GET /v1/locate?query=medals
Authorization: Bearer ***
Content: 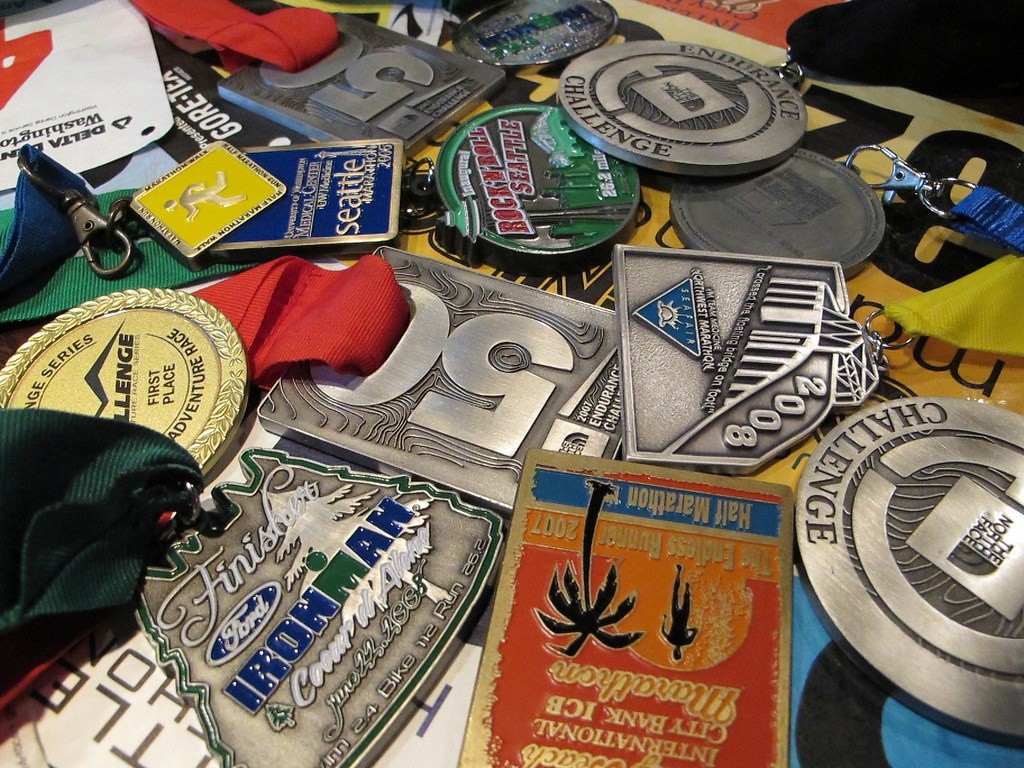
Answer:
[0,0,1024,768]
